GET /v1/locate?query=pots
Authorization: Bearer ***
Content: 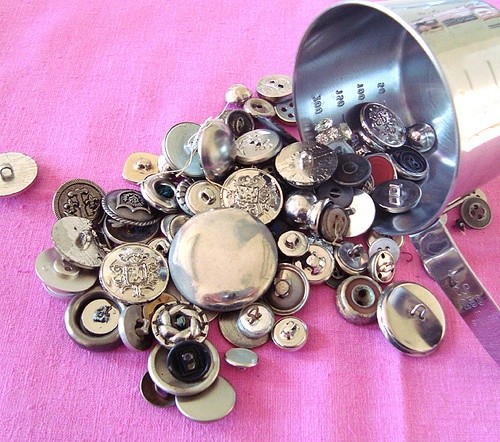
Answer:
[293,0,500,365]
